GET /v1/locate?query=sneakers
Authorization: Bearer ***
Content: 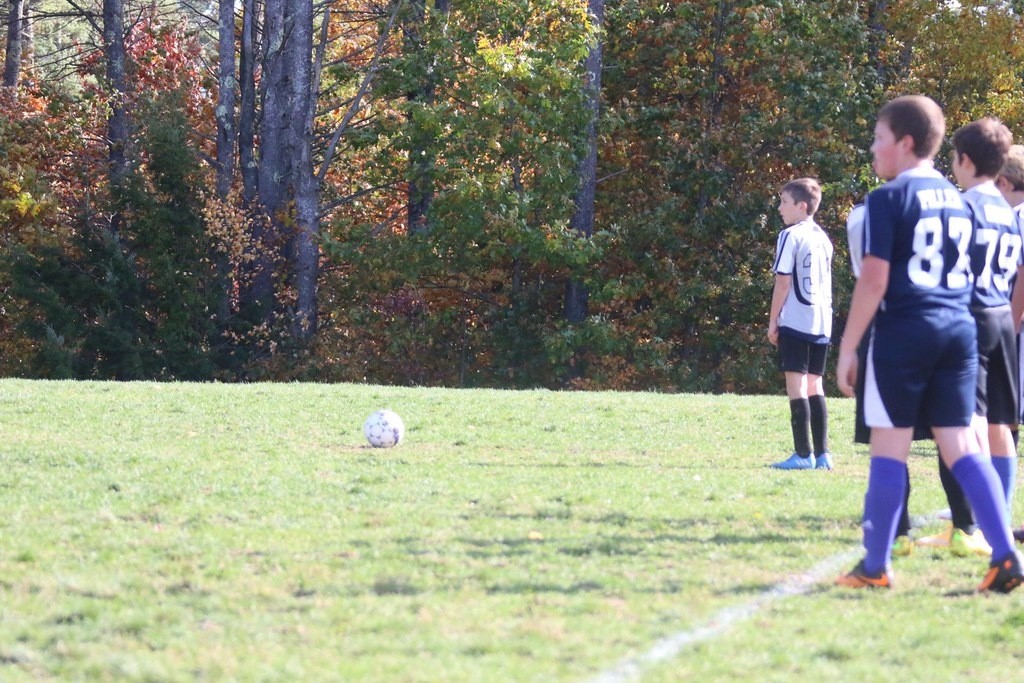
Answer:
[833,558,891,587]
[914,525,993,555]
[770,452,816,468]
[892,535,912,555]
[816,452,831,470]
[974,549,1024,593]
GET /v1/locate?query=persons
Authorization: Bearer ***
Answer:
[844,175,1003,562]
[931,142,1024,545]
[828,93,1024,595]
[763,177,836,472]
[913,114,1024,552]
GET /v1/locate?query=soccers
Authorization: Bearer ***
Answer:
[363,407,407,450]
